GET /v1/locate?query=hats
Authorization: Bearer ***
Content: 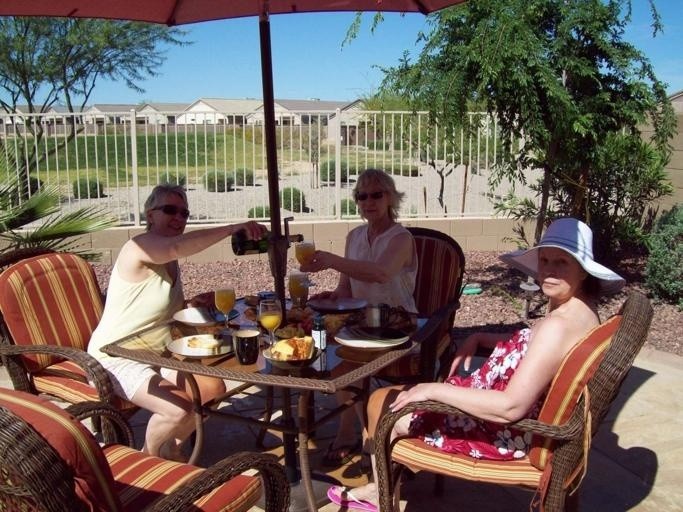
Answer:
[498,217,627,297]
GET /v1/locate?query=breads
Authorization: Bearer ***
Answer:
[271,336,316,362]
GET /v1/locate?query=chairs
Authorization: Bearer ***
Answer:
[373,292,654,512]
[0,385,291,512]
[368,227,465,383]
[0,246,198,449]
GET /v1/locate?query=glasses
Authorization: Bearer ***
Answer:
[355,192,383,200]
[152,205,190,219]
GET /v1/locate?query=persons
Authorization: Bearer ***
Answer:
[297,168,421,461]
[85,184,267,463]
[328,215,635,511]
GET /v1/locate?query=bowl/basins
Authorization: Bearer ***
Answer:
[262,347,324,369]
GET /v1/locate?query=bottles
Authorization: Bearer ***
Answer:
[310,317,327,351]
[231,231,304,256]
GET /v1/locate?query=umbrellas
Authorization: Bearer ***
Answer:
[0,1,461,483]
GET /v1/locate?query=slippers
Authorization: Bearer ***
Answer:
[327,486,378,511]
[320,442,351,465]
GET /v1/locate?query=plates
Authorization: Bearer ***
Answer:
[306,295,367,310]
[172,307,239,326]
[166,333,233,358]
[333,326,409,349]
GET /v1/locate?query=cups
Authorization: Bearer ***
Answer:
[244,295,258,307]
[364,301,383,327]
[288,273,308,306]
[233,330,260,364]
[244,308,256,322]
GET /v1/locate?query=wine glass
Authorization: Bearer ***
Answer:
[294,240,318,288]
[214,288,235,334]
[259,298,282,348]
